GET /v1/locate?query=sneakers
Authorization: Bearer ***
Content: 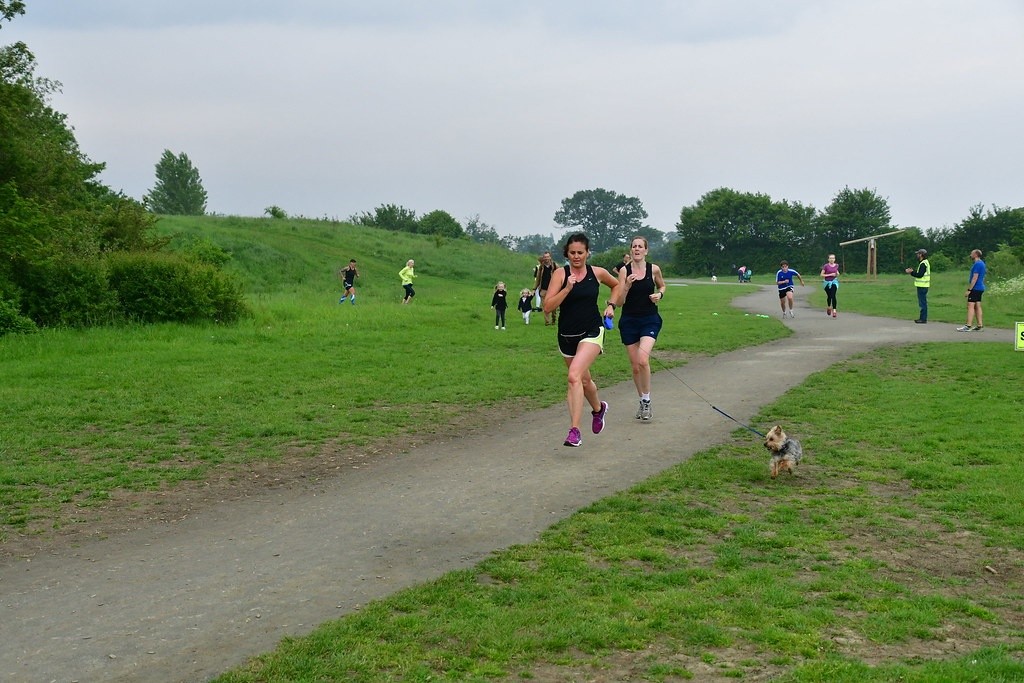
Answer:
[591,401,608,434]
[636,401,642,419]
[641,399,653,420]
[957,325,972,332]
[972,326,984,331]
[564,427,582,447]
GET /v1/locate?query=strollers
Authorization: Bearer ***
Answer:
[742,270,752,283]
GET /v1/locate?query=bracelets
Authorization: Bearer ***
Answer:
[968,288,972,291]
[658,291,664,299]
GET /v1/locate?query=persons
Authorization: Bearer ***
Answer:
[956,250,986,332]
[490,281,508,330]
[544,233,619,447]
[517,288,535,325]
[398,259,418,304]
[775,260,805,319]
[615,237,665,420]
[820,254,840,318]
[532,256,543,309]
[338,259,359,305]
[612,253,630,275]
[905,249,931,323]
[738,266,746,282]
[531,252,559,326]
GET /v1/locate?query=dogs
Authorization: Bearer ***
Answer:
[711,275,717,282]
[764,425,802,478]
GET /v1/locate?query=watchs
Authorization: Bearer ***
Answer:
[609,303,616,309]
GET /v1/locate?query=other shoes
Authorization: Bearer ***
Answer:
[833,312,836,317]
[496,326,499,329]
[789,310,795,318]
[551,321,556,325]
[501,327,506,330]
[545,322,549,325]
[783,311,787,319]
[827,308,831,315]
[915,319,927,323]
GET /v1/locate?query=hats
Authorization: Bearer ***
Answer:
[350,259,356,262]
[915,249,928,256]
